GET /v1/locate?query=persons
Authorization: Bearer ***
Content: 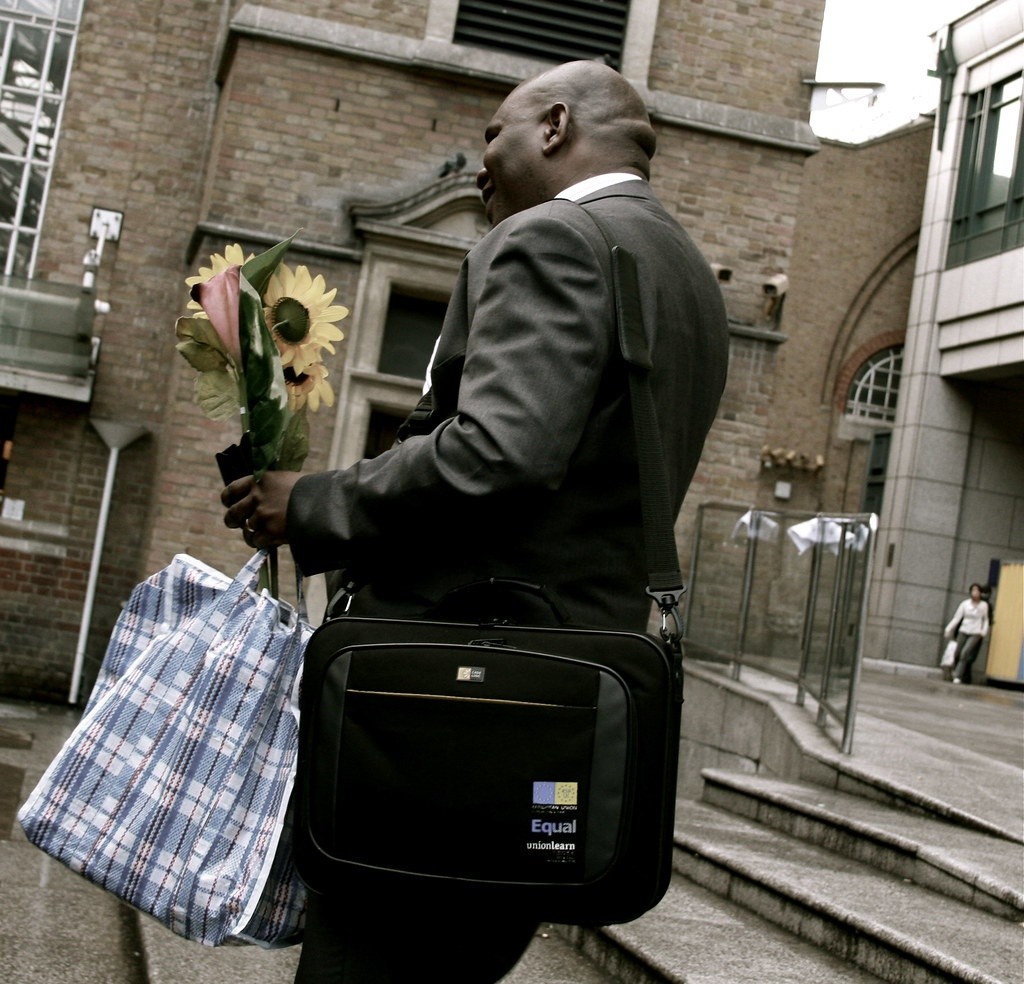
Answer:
[944,583,990,687]
[215,63,728,984]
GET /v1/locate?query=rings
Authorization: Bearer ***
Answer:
[242,519,256,536]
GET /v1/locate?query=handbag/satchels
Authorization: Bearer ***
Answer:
[15,544,329,952]
[289,606,685,930]
[938,636,961,670]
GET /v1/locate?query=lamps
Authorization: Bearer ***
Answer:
[758,440,826,499]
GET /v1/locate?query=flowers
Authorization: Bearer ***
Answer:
[172,229,350,599]
